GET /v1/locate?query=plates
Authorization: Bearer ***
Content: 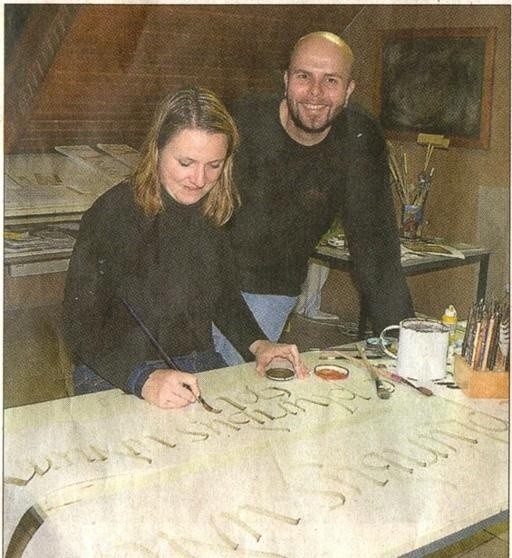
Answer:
[367,337,391,348]
[266,367,294,381]
[314,364,349,380]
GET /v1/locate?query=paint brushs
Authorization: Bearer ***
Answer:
[309,343,433,399]
[385,139,434,205]
[117,292,213,412]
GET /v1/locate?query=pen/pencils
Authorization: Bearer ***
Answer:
[461,298,502,372]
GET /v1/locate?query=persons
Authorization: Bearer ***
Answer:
[213,30,415,366]
[60,87,311,408]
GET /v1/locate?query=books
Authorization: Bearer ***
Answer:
[5,138,140,261]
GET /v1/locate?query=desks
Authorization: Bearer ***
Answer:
[2,330,510,557]
[310,227,493,337]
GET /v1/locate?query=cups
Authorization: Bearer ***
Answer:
[379,318,450,382]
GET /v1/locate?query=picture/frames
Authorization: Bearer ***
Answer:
[370,25,494,154]
